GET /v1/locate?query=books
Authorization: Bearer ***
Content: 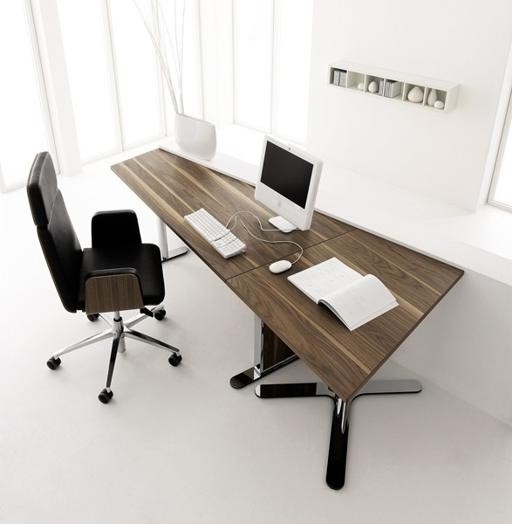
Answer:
[288,255,399,331]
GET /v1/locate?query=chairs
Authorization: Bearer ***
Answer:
[169,112,218,163]
[27,149,183,406]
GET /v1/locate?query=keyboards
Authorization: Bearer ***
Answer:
[184,208,247,260]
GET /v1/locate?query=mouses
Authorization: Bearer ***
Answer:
[269,260,292,273]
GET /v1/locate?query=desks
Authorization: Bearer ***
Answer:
[111,147,464,491]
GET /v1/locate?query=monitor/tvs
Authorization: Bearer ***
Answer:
[255,135,323,234]
